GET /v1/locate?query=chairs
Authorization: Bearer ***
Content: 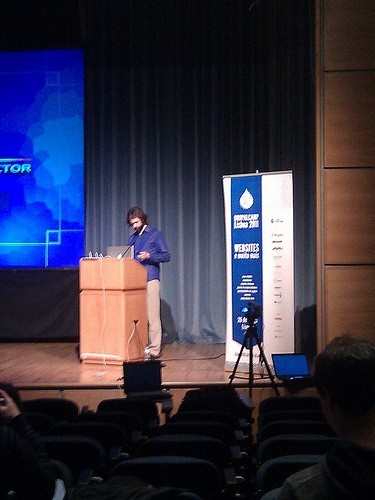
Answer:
[14,391,341,500]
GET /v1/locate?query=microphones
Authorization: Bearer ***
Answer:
[121,242,135,259]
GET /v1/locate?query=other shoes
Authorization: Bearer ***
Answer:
[144,353,157,360]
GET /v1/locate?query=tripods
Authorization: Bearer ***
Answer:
[228,318,280,399]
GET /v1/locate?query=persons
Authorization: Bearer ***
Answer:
[0,381,56,500]
[67,475,185,500]
[276,333,375,500]
[126,207,171,357]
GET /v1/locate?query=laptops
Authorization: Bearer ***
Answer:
[123,360,173,399]
[108,246,134,259]
[271,353,314,381]
